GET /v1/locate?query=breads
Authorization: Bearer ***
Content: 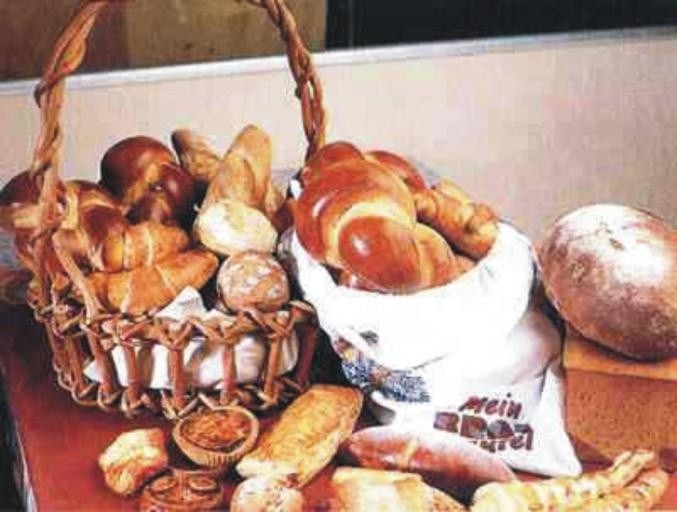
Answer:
[173,405,258,467]
[329,465,464,511]
[539,204,676,360]
[0,124,290,322]
[140,472,224,511]
[230,476,307,512]
[293,142,495,296]
[236,383,363,487]
[471,447,669,512]
[98,428,166,495]
[344,424,516,503]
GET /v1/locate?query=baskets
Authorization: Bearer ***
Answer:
[1,0,328,420]
